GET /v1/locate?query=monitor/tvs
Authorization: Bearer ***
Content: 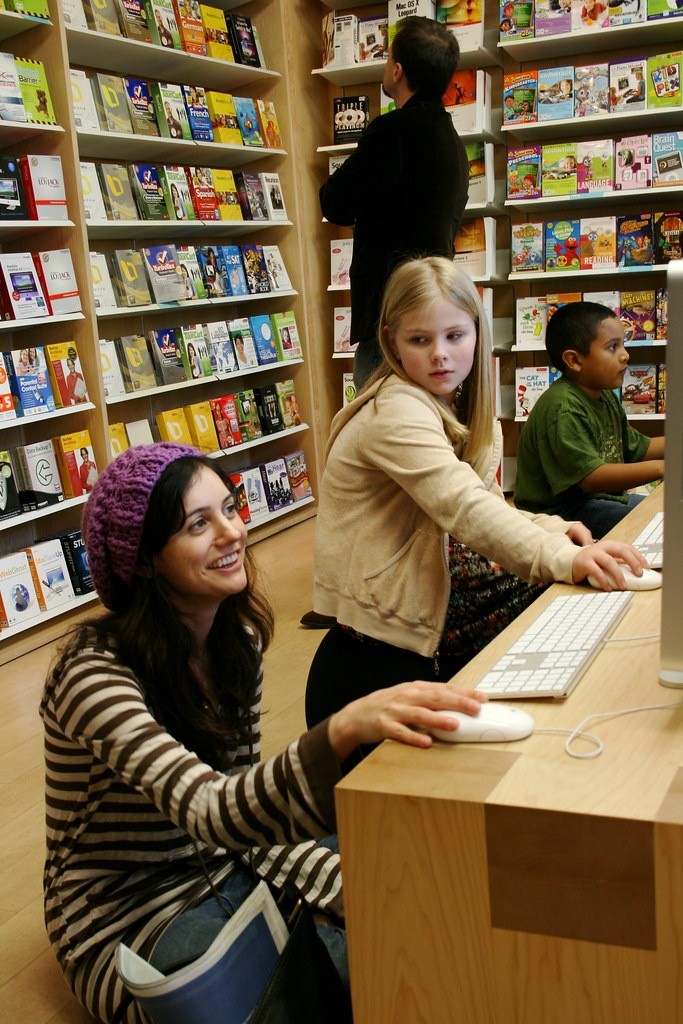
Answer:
[655,259,683,691]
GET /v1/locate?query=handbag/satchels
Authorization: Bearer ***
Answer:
[112,860,352,1024]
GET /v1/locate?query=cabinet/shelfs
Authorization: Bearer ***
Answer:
[311,0,683,504]
[0,0,324,661]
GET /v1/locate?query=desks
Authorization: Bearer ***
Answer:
[335,481,683,1023]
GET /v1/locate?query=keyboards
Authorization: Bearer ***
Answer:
[477,589,636,699]
[613,512,666,569]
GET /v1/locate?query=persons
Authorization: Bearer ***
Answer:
[194,167,214,189]
[188,85,202,108]
[80,446,99,493]
[509,299,667,539]
[153,8,174,49]
[179,264,198,300]
[272,185,277,208]
[280,327,292,349]
[169,342,183,363]
[233,332,250,367]
[38,443,488,1024]
[27,348,40,370]
[66,357,89,404]
[625,72,645,104]
[558,78,572,98]
[283,398,296,425]
[609,87,621,106]
[319,13,471,405]
[166,101,183,138]
[170,184,189,220]
[313,251,648,660]
[205,246,226,296]
[289,393,299,415]
[18,349,30,376]
[186,343,204,378]
[246,393,261,431]
[563,155,577,170]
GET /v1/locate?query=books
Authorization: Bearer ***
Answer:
[501,0,683,424]
[0,0,320,632]
[317,0,487,408]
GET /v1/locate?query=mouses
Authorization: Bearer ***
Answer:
[587,561,662,592]
[427,701,537,742]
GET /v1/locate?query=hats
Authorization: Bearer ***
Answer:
[80,442,205,612]
